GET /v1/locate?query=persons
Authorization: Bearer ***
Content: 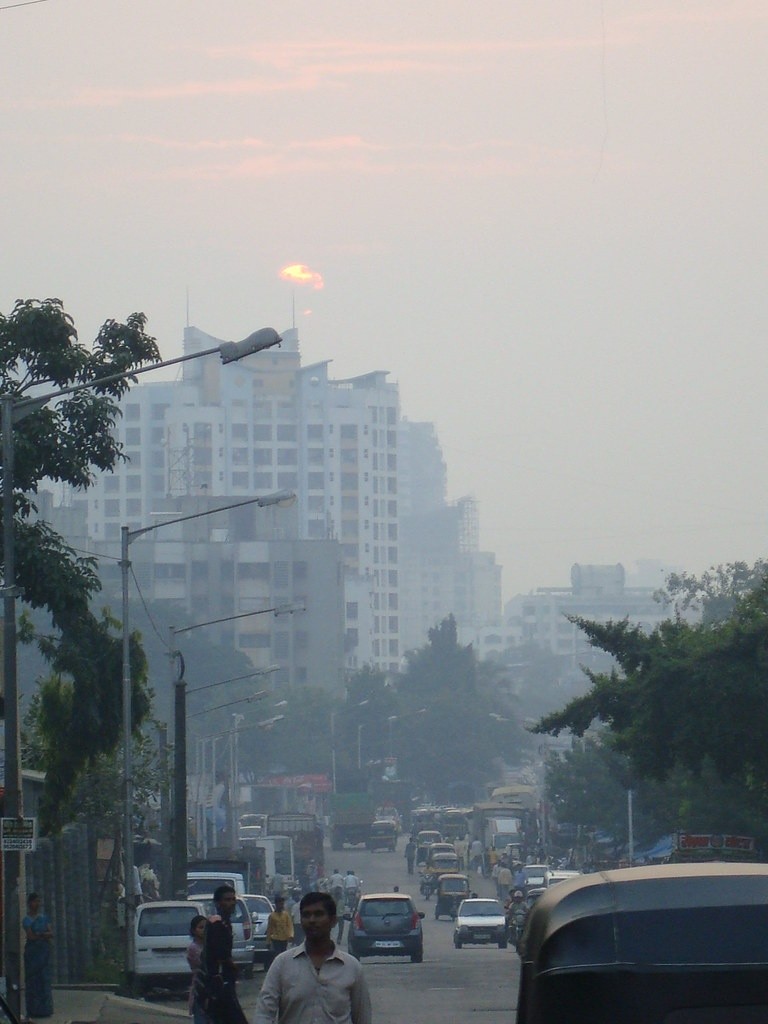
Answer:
[318,869,358,911]
[252,893,374,1024]
[185,884,250,1024]
[505,889,529,932]
[521,840,598,875]
[22,893,54,1016]
[265,893,304,952]
[453,829,527,901]
[404,837,416,874]
[265,869,286,899]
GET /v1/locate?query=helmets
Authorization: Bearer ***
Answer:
[514,891,523,902]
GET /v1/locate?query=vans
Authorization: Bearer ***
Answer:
[523,864,583,906]
[453,898,507,949]
[133,901,209,975]
[186,893,258,966]
[239,894,275,954]
[186,872,245,894]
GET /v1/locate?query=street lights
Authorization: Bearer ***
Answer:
[487,713,585,751]
[2,324,284,1022]
[509,649,634,861]
[169,602,305,863]
[118,490,298,991]
[332,698,427,792]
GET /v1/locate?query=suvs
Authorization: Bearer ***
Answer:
[343,893,426,963]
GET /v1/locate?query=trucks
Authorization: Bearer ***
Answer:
[473,784,539,847]
[331,792,375,851]
[267,812,325,876]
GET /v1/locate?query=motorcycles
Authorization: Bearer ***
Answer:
[368,819,397,854]
[411,806,473,920]
[516,862,768,1024]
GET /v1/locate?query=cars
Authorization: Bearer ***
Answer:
[376,807,402,823]
